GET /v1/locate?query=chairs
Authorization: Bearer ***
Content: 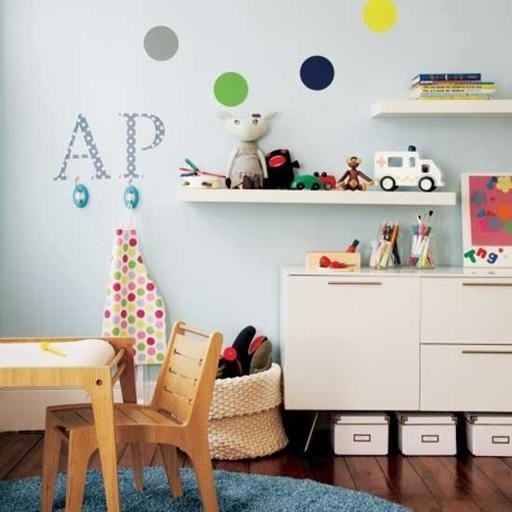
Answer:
[40,320,224,512]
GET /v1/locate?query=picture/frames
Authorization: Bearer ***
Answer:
[460,173,512,266]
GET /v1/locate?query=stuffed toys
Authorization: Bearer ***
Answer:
[262,150,300,188]
[336,155,374,191]
[216,110,277,189]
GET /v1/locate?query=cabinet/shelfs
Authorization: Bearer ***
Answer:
[279,265,512,413]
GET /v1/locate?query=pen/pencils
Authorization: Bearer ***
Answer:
[41,341,67,358]
[411,208,435,270]
[373,218,401,269]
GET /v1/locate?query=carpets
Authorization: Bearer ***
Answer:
[0,464,417,512]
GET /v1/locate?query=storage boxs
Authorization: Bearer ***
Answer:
[465,413,511,458]
[328,414,390,457]
[397,414,459,457]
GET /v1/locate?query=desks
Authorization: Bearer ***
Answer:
[1,334,144,512]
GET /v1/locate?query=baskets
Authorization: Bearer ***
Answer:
[208,363,290,460]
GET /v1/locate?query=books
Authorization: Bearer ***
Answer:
[410,73,496,101]
